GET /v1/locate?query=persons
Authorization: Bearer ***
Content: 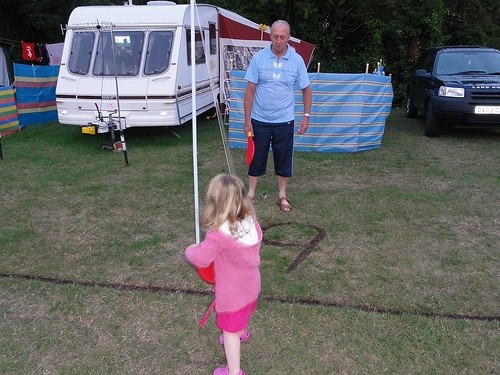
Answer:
[244,20,312,212]
[184,173,264,375]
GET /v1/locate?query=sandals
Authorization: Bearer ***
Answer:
[213,367,245,375]
[217,331,250,344]
[277,197,292,212]
[248,194,254,201]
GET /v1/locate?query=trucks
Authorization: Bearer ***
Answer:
[55,1,218,128]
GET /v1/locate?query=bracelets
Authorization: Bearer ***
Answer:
[304,113,310,118]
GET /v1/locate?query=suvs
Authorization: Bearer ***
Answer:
[406,45,500,138]
